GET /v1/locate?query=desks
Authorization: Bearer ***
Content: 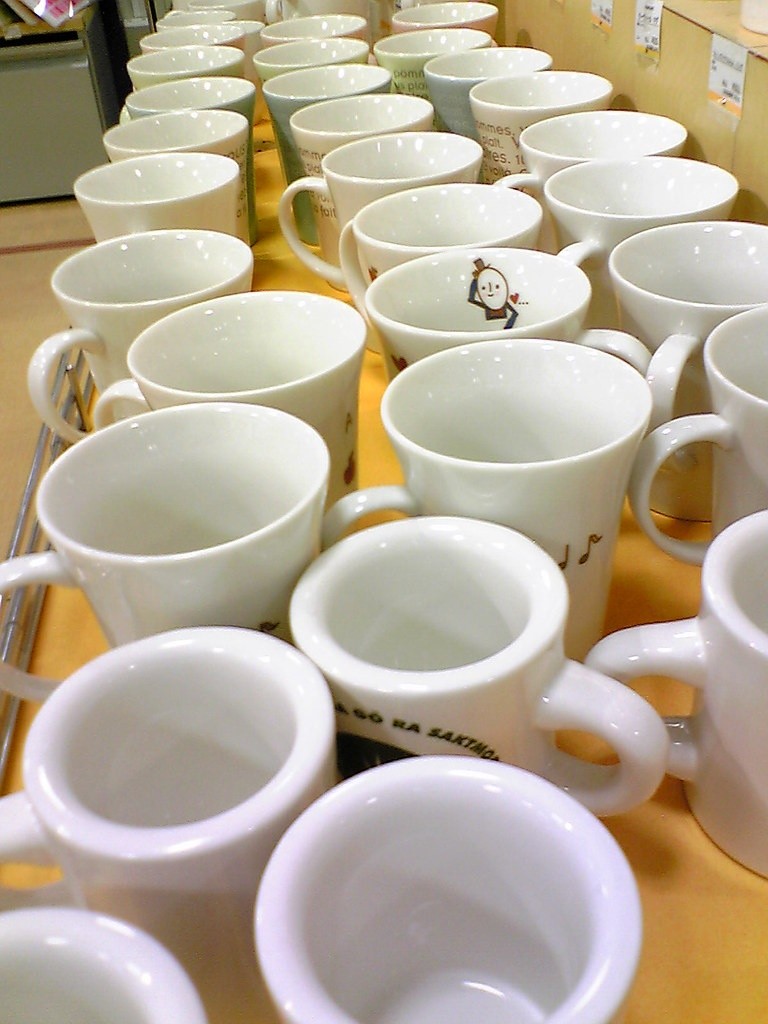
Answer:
[0,77,768,1024]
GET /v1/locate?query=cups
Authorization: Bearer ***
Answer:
[0,628,339,1024]
[0,400,330,702]
[627,306,768,567]
[287,515,671,818]
[587,508,768,880]
[252,754,642,1024]
[26,0,768,506]
[323,339,654,665]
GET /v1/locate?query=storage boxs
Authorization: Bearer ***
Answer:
[502,0,768,226]
